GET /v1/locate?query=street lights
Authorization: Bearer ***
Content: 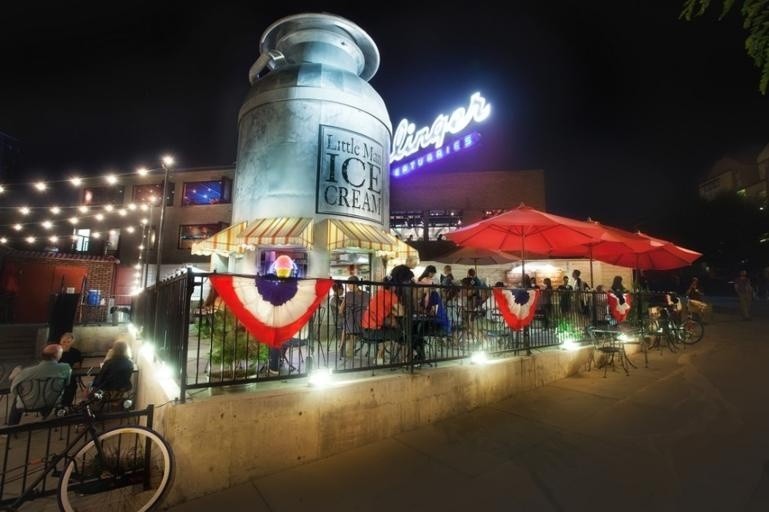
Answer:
[136,154,174,292]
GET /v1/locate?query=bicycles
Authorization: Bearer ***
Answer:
[635,292,705,350]
[0,390,176,512]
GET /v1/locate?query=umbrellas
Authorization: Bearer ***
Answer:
[596,227,705,328]
[548,216,664,330]
[440,201,625,358]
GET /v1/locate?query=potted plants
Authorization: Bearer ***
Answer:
[192,308,269,397]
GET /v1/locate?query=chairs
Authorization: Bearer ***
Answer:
[279,285,686,386]
[1,346,142,441]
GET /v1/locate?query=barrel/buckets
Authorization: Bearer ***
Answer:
[87,290,101,305]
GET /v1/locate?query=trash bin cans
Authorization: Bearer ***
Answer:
[110,306,129,325]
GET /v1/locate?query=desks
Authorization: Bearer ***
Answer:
[74,302,106,326]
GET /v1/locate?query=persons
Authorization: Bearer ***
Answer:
[407,234,412,242]
[9,333,133,426]
[687,277,699,301]
[524,269,627,327]
[331,264,482,365]
[734,268,753,320]
[438,234,442,240]
